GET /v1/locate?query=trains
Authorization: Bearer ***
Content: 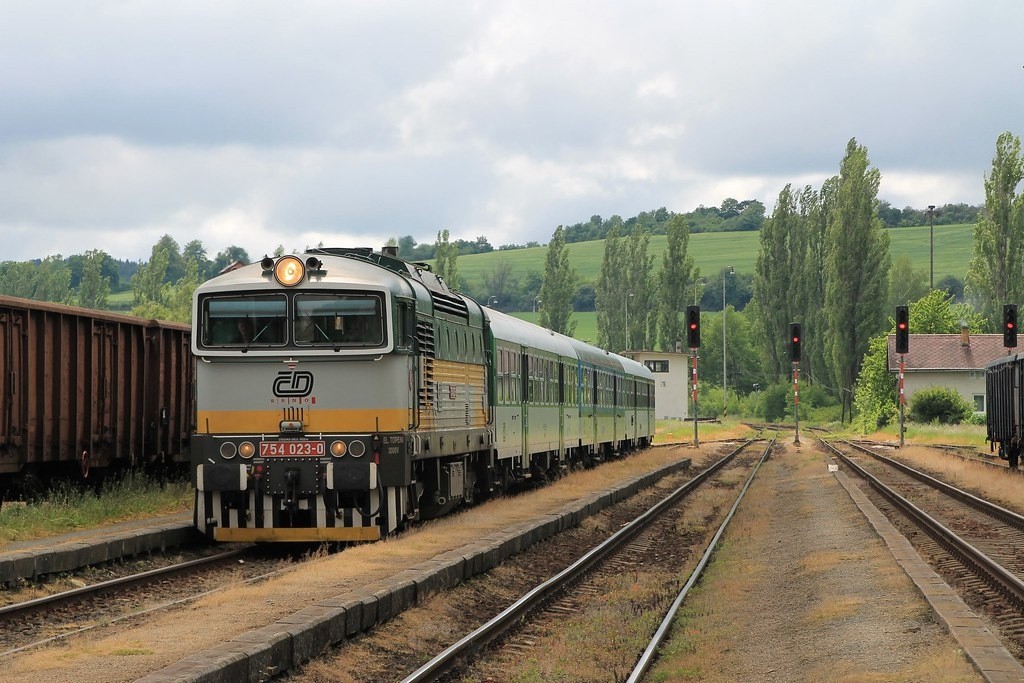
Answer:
[188,246,658,555]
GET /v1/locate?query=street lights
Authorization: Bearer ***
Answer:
[625,287,637,351]
[695,277,708,305]
[722,264,736,424]
[533,295,543,324]
[487,294,501,308]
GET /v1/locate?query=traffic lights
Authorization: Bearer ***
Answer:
[790,323,802,363]
[895,306,908,353]
[1003,303,1018,348]
[686,306,701,347]
